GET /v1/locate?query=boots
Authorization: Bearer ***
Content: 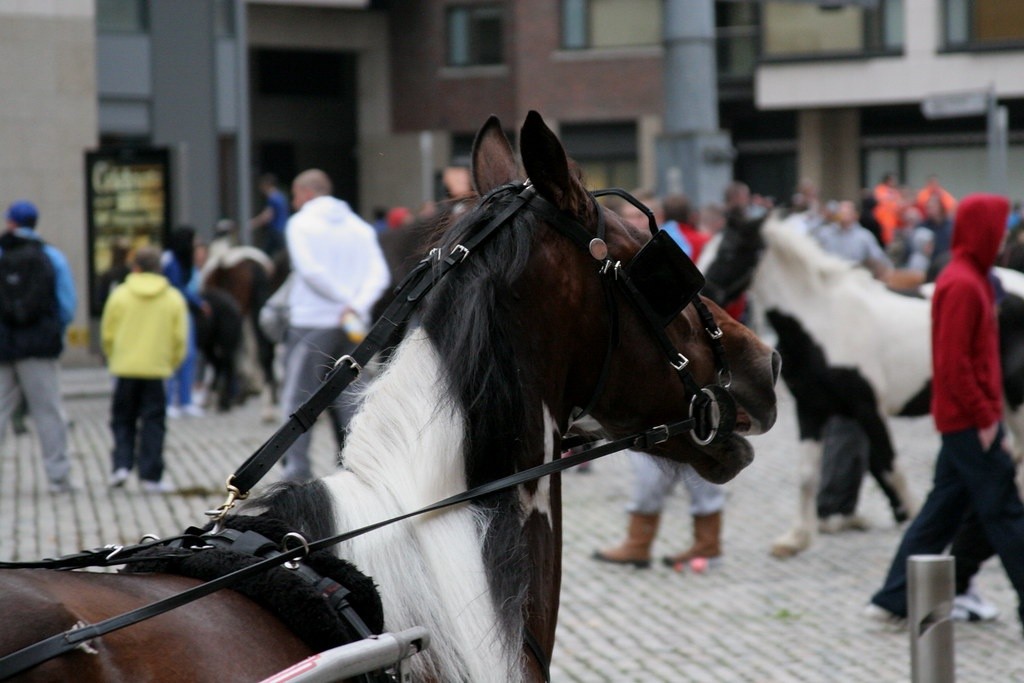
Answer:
[661,512,721,566]
[593,510,661,570]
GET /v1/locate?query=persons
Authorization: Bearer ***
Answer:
[99,246,188,494]
[98,168,1024,637]
[276,168,393,485]
[0,198,80,496]
[865,193,1024,636]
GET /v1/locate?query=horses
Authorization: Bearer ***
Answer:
[0,107,784,682]
[694,200,1024,559]
[180,240,288,416]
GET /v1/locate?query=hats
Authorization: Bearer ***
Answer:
[8,202,39,230]
[135,246,161,274]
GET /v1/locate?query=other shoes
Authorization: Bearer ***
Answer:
[105,469,127,489]
[952,593,999,626]
[862,602,908,632]
[137,477,172,492]
[167,406,198,418]
[51,479,81,497]
